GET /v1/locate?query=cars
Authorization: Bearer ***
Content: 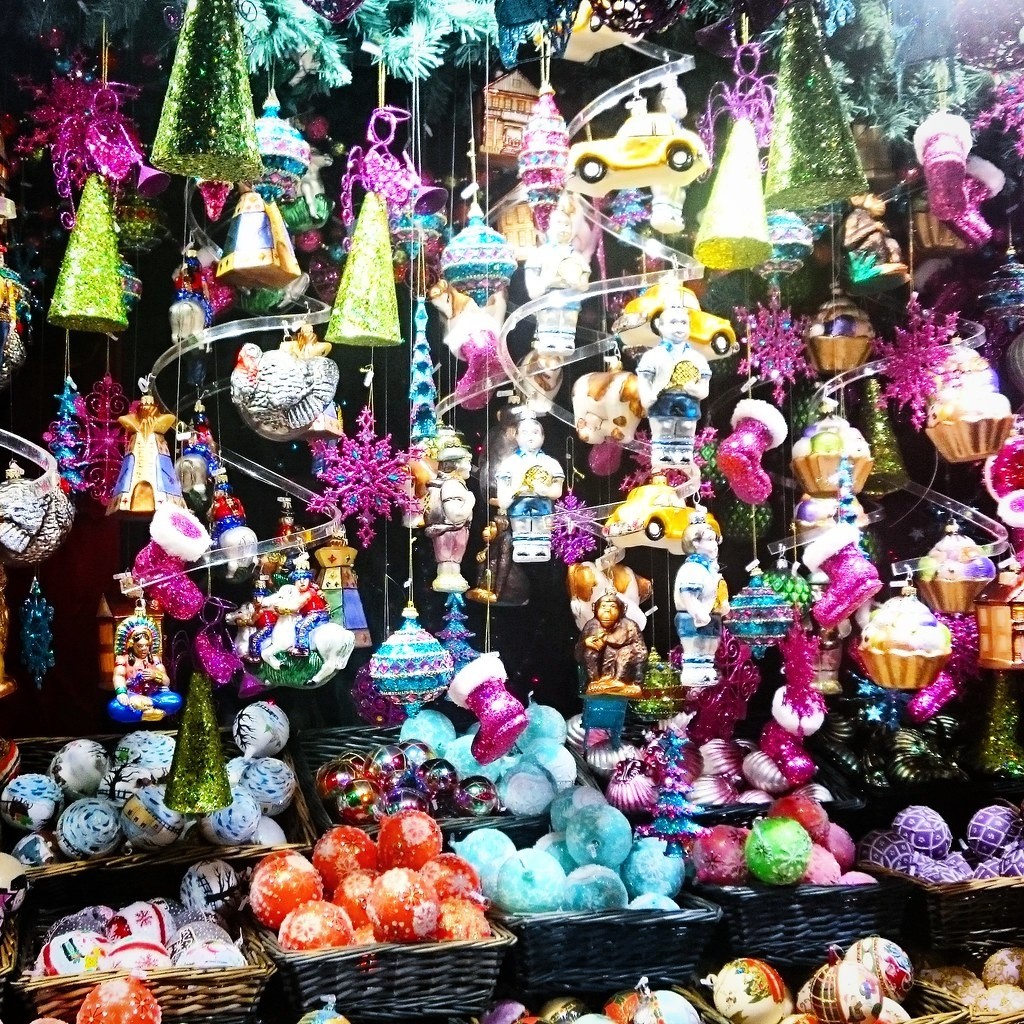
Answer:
[605,477,727,553]
[570,111,703,182]
[615,279,743,360]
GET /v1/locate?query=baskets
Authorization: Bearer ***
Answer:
[0,725,1024,1024]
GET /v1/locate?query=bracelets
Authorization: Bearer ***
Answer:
[116,687,126,694]
[154,673,162,683]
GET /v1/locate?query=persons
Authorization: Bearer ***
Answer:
[109,628,182,722]
[430,446,475,593]
[673,523,731,688]
[184,413,218,482]
[639,308,713,467]
[273,570,330,657]
[173,257,212,329]
[237,588,278,664]
[523,210,591,356]
[278,509,299,571]
[206,482,245,550]
[498,417,565,561]
